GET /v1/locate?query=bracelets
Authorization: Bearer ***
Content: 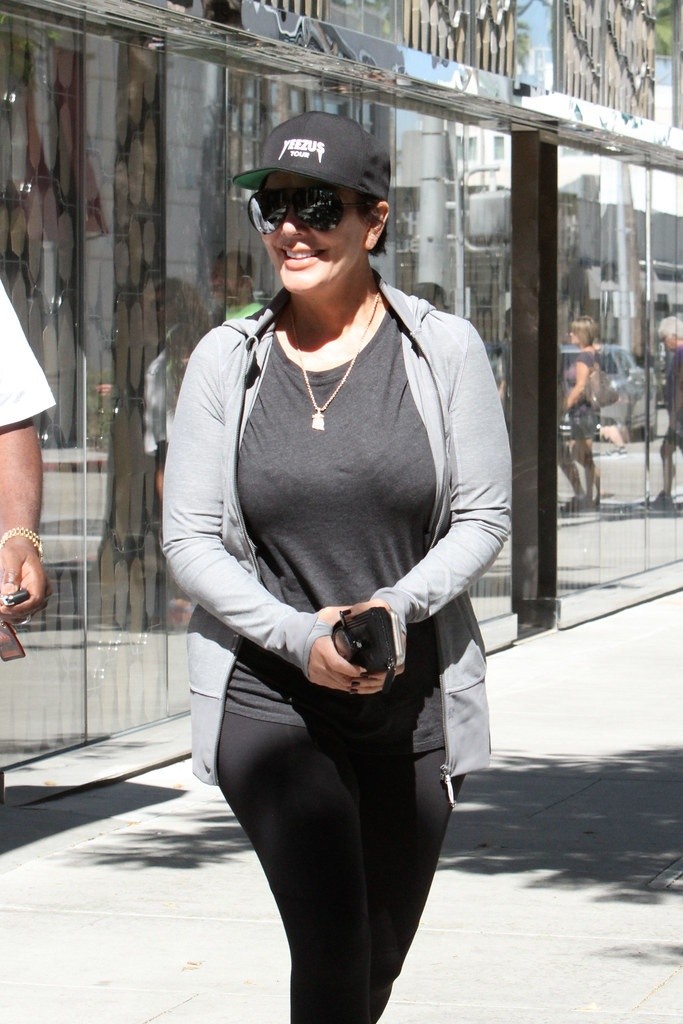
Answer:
[0,526,43,563]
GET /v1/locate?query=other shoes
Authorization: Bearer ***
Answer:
[559,493,596,513]
[639,492,675,512]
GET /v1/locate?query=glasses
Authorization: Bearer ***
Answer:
[142,295,172,320]
[332,610,356,664]
[248,188,375,235]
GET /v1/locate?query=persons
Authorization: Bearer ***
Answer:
[0,275,56,627]
[497,306,627,510]
[212,248,265,324]
[649,315,683,511]
[141,324,198,628]
[164,112,511,1024]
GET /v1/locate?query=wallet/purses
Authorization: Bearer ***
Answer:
[333,606,396,684]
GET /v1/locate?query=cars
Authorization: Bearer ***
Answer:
[558,341,658,449]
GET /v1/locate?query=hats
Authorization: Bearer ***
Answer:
[232,111,391,201]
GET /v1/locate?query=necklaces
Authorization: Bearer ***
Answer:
[289,293,380,431]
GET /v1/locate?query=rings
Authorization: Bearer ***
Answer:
[26,614,31,623]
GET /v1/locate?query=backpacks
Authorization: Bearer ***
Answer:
[585,368,620,411]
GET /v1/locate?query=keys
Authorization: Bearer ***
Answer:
[0,590,29,663]
